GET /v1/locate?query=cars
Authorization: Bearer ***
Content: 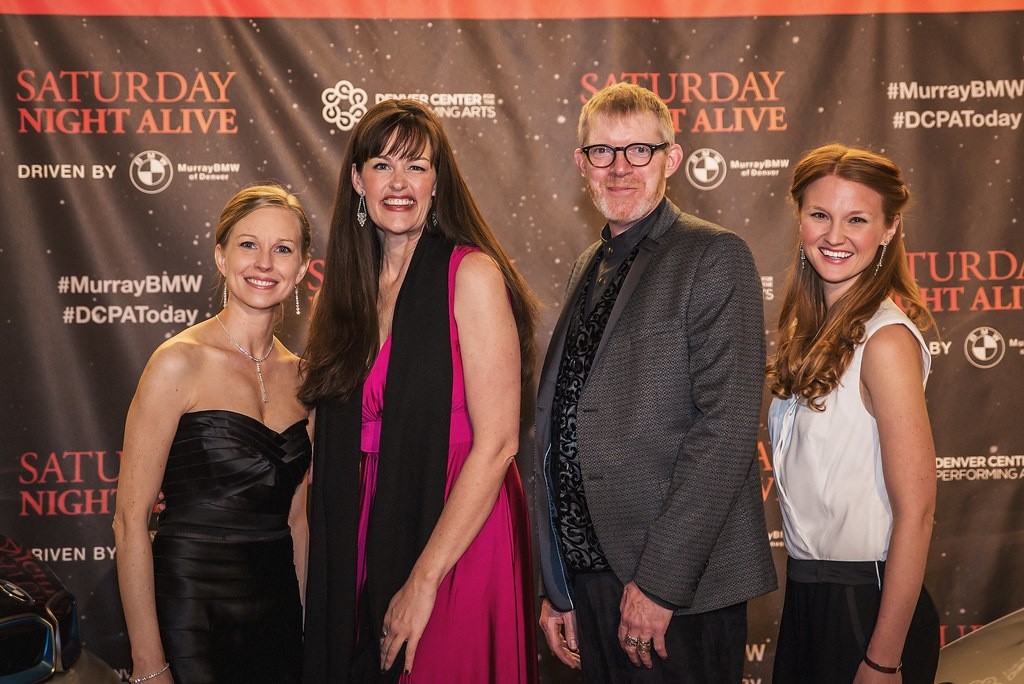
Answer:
[0,534,126,684]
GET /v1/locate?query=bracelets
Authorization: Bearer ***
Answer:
[863,653,902,674]
[129,662,171,684]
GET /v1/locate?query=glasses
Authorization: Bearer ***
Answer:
[580,141,669,168]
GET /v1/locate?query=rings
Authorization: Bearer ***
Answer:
[637,642,651,653]
[624,634,638,647]
[379,630,388,640]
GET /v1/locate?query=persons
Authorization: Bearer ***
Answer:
[290,98,539,684]
[111,182,315,683]
[757,142,943,683]
[532,83,780,684]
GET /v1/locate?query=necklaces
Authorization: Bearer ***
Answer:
[214,314,275,405]
